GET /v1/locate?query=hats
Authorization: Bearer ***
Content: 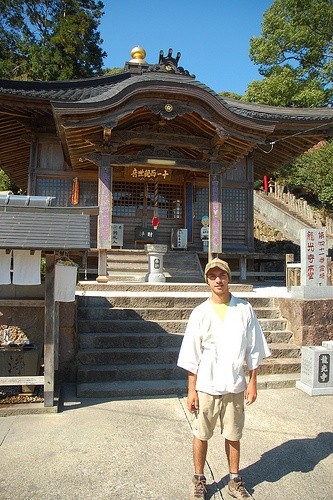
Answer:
[204,258,231,282]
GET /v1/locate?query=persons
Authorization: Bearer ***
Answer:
[175,257,273,500]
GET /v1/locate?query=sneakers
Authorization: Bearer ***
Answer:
[191,475,206,500]
[228,476,255,500]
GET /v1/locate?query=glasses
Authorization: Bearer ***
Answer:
[208,273,227,281]
